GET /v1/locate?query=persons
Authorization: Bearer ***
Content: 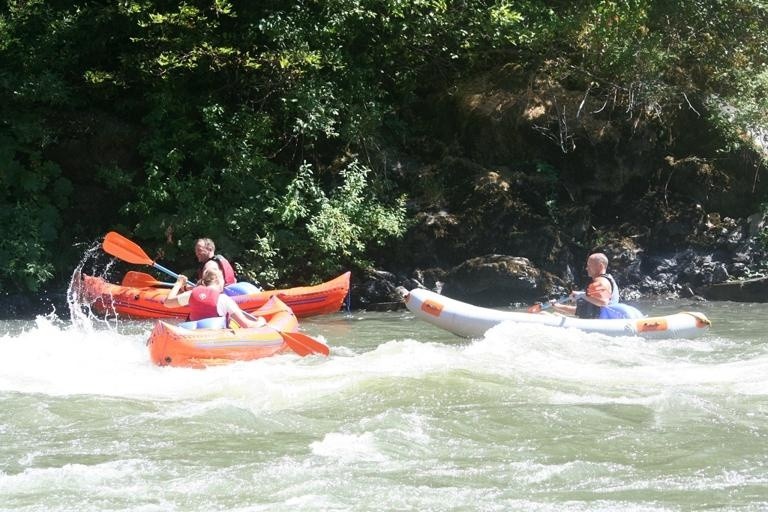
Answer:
[195,238,238,286]
[164,268,266,330]
[539,253,619,319]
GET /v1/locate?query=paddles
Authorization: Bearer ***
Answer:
[240,310,328,359]
[102,231,198,289]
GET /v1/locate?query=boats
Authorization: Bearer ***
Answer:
[82,269,351,321]
[403,286,712,343]
[147,295,300,368]
